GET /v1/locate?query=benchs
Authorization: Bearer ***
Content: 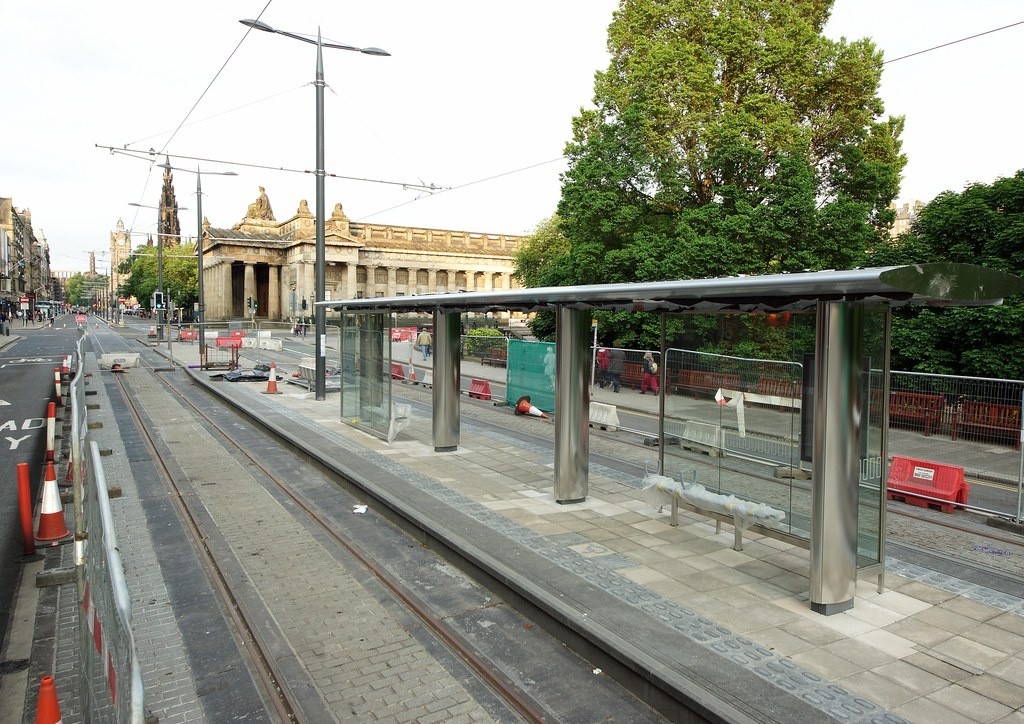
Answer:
[641,459,785,551]
[363,400,408,439]
[481,349,1022,452]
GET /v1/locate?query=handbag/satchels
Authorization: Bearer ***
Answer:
[297,327,302,331]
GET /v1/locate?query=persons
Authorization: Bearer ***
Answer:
[543,347,557,390]
[593,342,625,393]
[246,185,276,220]
[0,307,55,326]
[639,352,659,395]
[289,313,308,337]
[417,328,432,361]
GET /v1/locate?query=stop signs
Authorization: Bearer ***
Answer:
[21,297,29,303]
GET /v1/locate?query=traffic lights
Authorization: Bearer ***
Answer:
[302,300,306,309]
[247,297,251,308]
[254,301,257,308]
[154,292,163,309]
[6,301,11,307]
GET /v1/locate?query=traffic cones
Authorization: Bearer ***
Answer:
[34,461,70,541]
[148,323,156,338]
[514,396,549,419]
[408,359,416,381]
[63,323,67,329]
[111,364,128,373]
[34,676,63,724]
[261,361,283,394]
[95,322,99,329]
[62,355,70,374]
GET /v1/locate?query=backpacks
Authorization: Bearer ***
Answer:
[646,358,657,374]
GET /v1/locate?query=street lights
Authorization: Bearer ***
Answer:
[129,203,189,339]
[240,19,392,401]
[156,163,239,354]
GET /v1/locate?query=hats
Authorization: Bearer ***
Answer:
[422,328,427,330]
[547,347,552,351]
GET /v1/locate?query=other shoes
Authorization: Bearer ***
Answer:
[608,381,613,389]
[614,386,620,393]
[639,392,645,394]
[426,355,427,356]
[655,390,658,396]
[597,386,605,390]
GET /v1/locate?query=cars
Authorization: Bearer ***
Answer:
[79,308,86,315]
